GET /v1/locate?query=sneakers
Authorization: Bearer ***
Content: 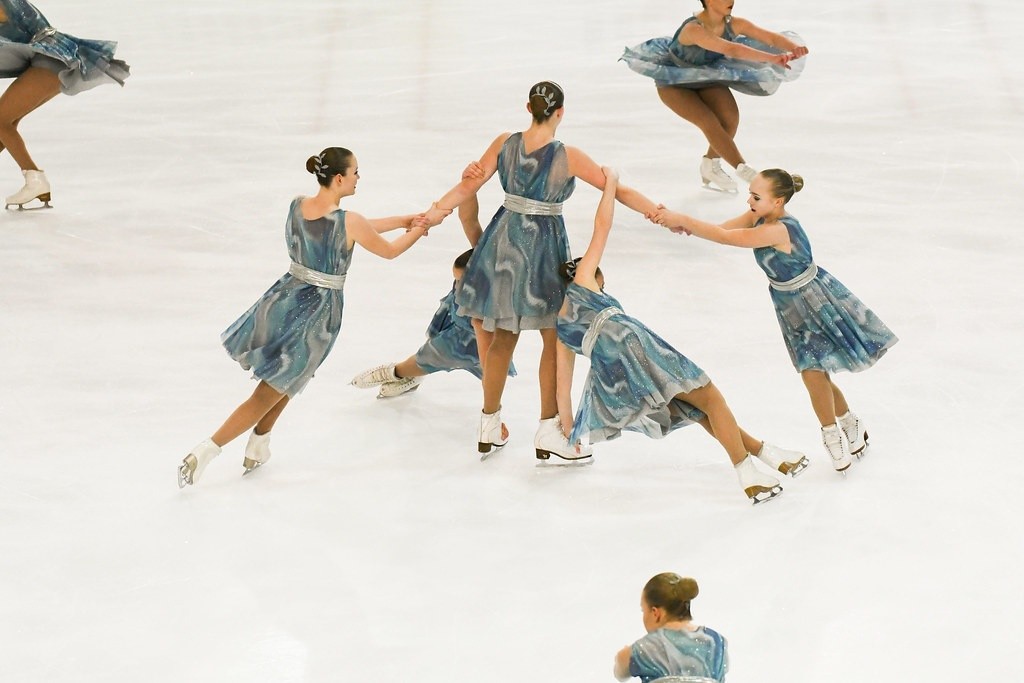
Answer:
[700,155,757,194]
[347,363,424,399]
[243,427,270,475]
[734,452,783,504]
[6,169,51,210]
[534,413,592,460]
[821,424,851,476]
[479,405,509,452]
[178,437,221,488]
[835,409,868,459]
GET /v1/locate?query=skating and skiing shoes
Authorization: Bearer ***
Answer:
[757,441,812,477]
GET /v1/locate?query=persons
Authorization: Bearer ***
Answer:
[458,82,691,466]
[179,147,452,488]
[347,161,517,400]
[617,0,808,193]
[556,166,809,505]
[0,0,130,211]
[645,168,898,476]
[614,572,729,683]
